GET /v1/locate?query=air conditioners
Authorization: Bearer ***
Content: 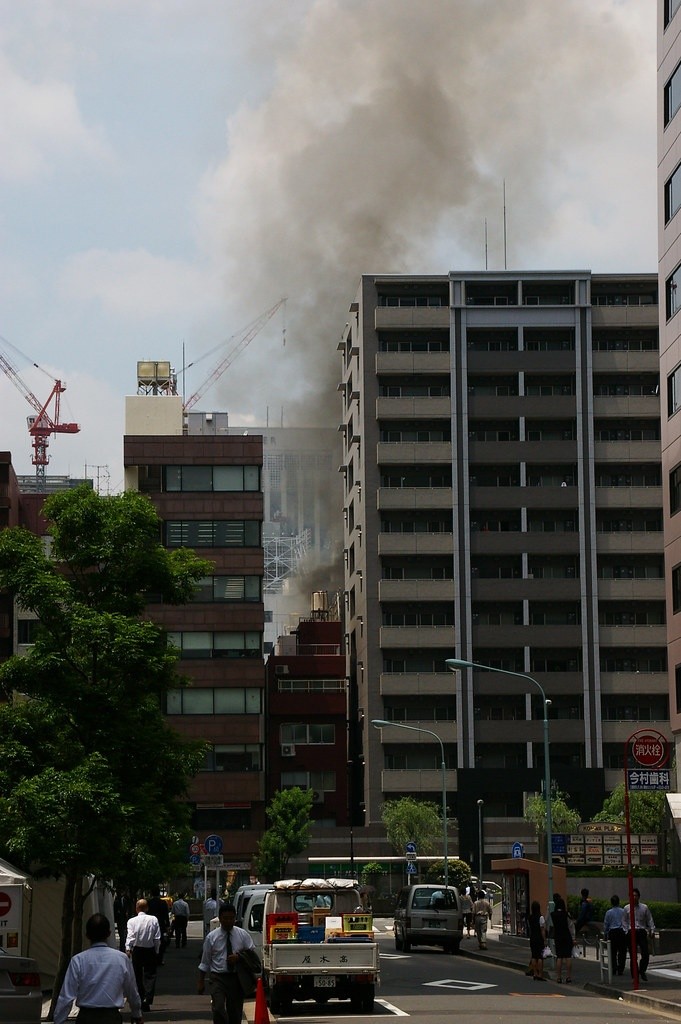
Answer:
[312,790,324,802]
[275,665,288,674]
[281,744,295,756]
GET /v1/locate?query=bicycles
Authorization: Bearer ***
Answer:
[570,909,600,946]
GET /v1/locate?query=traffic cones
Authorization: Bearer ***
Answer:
[254,977,270,1024]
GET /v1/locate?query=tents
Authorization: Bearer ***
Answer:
[0,859,35,957]
[26,873,114,977]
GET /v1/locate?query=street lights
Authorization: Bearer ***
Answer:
[370,720,448,886]
[476,799,484,890]
[444,659,556,956]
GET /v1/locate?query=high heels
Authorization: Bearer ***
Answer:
[537,976,547,981]
[533,975,538,980]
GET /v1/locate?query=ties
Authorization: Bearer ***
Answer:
[226,932,235,972]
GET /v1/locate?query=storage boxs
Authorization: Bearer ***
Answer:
[266,911,375,943]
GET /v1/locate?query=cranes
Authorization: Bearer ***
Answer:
[138,295,289,414]
[0,335,82,494]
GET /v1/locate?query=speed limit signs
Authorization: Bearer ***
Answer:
[190,844,200,854]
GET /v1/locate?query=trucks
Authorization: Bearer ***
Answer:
[261,887,380,1015]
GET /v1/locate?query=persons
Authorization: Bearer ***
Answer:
[470,887,477,904]
[459,888,475,938]
[198,905,256,1024]
[548,893,576,983]
[528,902,547,981]
[172,893,190,948]
[55,913,144,1024]
[125,899,161,1012]
[207,889,224,909]
[604,895,628,975]
[148,887,170,966]
[574,889,594,933]
[431,891,445,904]
[114,891,134,951]
[473,891,493,949]
[622,888,656,981]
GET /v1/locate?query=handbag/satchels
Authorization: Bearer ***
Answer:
[542,946,554,958]
[571,945,581,958]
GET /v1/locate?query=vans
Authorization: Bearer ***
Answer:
[232,885,275,971]
[392,884,464,953]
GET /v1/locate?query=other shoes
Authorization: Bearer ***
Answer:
[479,943,488,950]
[142,1002,150,1012]
[639,969,648,981]
[566,977,571,983]
[618,970,623,975]
[557,978,562,983]
[612,967,617,975]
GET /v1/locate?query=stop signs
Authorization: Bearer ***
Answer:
[0,892,12,917]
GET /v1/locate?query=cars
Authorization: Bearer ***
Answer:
[0,948,42,1024]
[437,874,502,892]
